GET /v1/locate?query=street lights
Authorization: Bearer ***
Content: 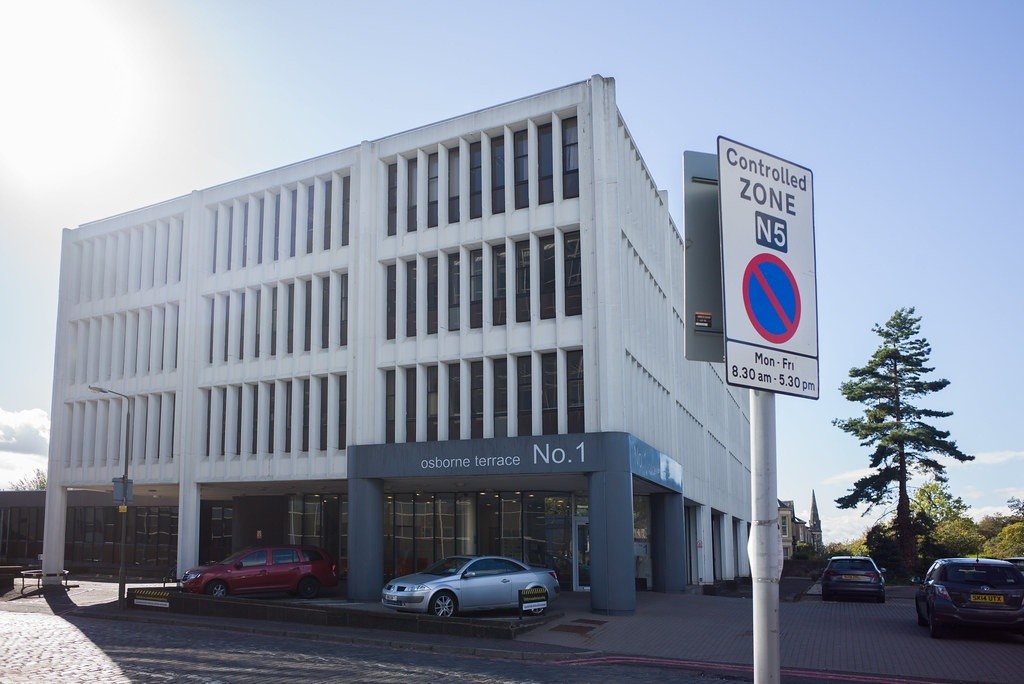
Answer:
[89,385,130,609]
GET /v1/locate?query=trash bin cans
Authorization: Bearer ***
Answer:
[635,577,647,592]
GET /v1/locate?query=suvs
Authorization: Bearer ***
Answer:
[179,545,337,598]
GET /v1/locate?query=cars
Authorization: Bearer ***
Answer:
[910,557,1024,639]
[822,555,885,604]
[503,550,591,591]
[381,556,562,617]
